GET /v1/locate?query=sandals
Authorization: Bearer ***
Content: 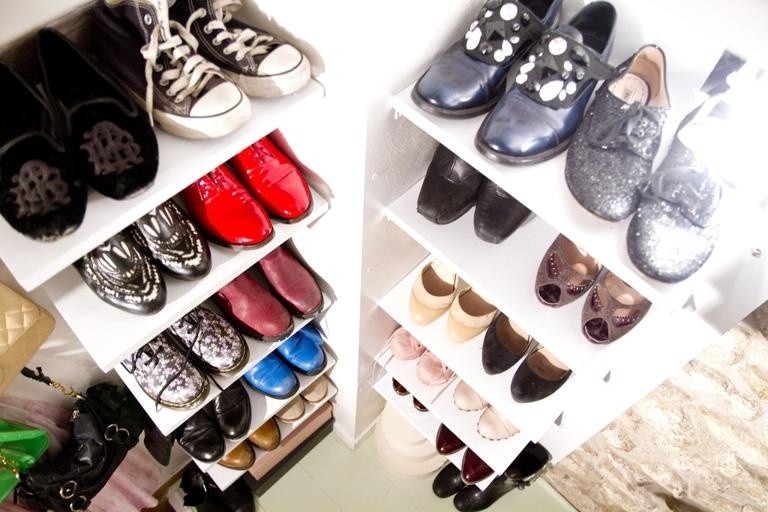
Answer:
[406,259,573,405]
[533,231,653,347]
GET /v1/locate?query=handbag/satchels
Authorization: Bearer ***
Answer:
[0,365,149,512]
[0,279,56,399]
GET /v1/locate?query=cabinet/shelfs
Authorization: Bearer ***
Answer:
[372,50,754,490]
[1,50,339,490]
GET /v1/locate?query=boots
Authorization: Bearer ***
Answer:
[431,439,550,512]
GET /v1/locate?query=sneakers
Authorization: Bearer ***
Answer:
[207,244,325,344]
[119,304,250,412]
[0,25,160,244]
[273,375,329,425]
[388,326,523,443]
[73,0,313,142]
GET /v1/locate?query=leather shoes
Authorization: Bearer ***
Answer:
[434,422,496,487]
[215,416,281,471]
[242,322,327,400]
[390,376,431,413]
[69,196,213,317]
[414,141,534,245]
[408,0,620,169]
[174,134,314,251]
[169,377,252,465]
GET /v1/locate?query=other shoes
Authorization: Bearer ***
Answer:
[564,44,671,221]
[627,94,744,283]
